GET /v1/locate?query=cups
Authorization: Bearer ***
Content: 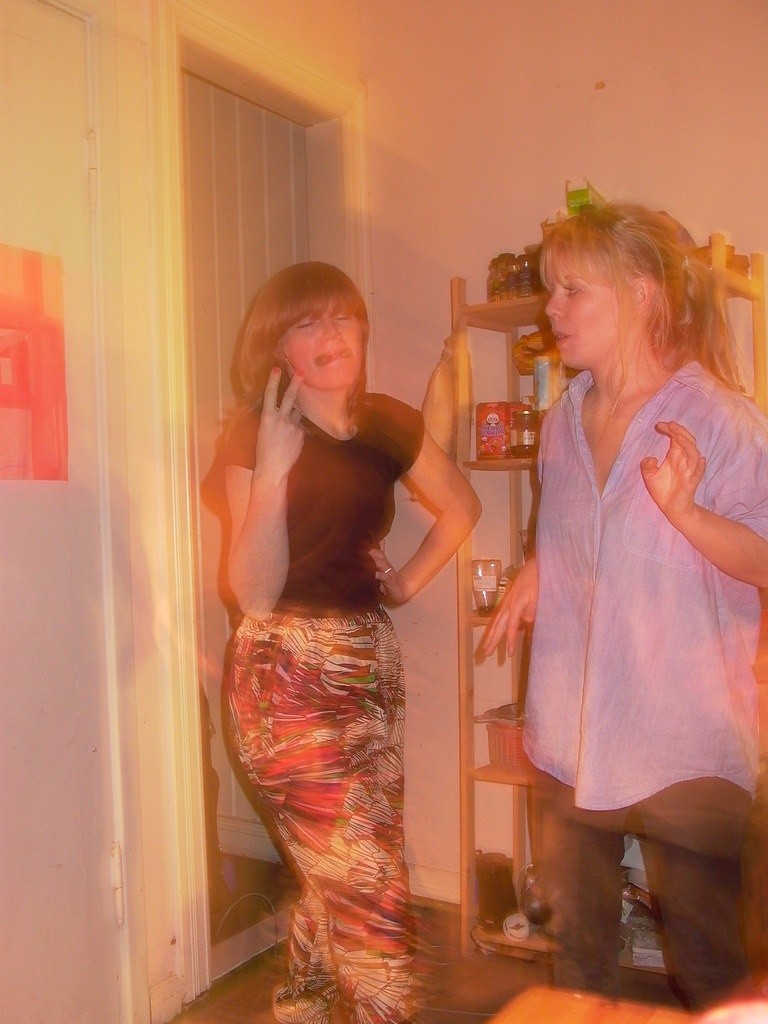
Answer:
[471,560,502,617]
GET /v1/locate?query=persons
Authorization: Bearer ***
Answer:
[228,262,484,1024]
[479,207,768,1013]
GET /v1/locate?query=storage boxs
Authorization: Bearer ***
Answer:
[566,178,611,217]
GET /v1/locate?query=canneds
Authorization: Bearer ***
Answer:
[509,410,541,458]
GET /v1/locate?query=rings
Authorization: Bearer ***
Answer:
[385,568,392,573]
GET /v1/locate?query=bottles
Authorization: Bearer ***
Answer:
[487,244,545,303]
[510,410,540,458]
[621,881,652,918]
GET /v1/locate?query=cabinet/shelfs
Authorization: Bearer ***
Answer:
[451,233,768,977]
